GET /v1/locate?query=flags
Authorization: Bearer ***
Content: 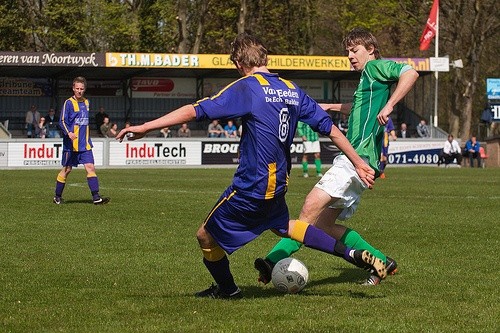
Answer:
[420,0,439,53]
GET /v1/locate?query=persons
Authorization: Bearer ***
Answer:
[115,37,388,299]
[24,104,62,138]
[464,136,482,168]
[52,76,111,206]
[255,30,419,288]
[338,113,349,135]
[397,123,412,139]
[95,107,134,140]
[442,135,462,166]
[416,119,430,138]
[296,113,324,179]
[379,117,397,180]
[158,120,242,139]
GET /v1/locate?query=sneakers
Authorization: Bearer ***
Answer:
[196,281,243,300]
[53,196,65,205]
[360,255,398,286]
[94,196,111,205]
[353,248,388,279]
[254,257,278,285]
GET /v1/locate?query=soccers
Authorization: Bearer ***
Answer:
[271,257,309,293]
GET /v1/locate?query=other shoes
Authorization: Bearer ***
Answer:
[304,174,308,178]
[380,172,385,179]
[318,173,323,177]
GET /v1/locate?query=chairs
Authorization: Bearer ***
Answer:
[442,147,488,168]
